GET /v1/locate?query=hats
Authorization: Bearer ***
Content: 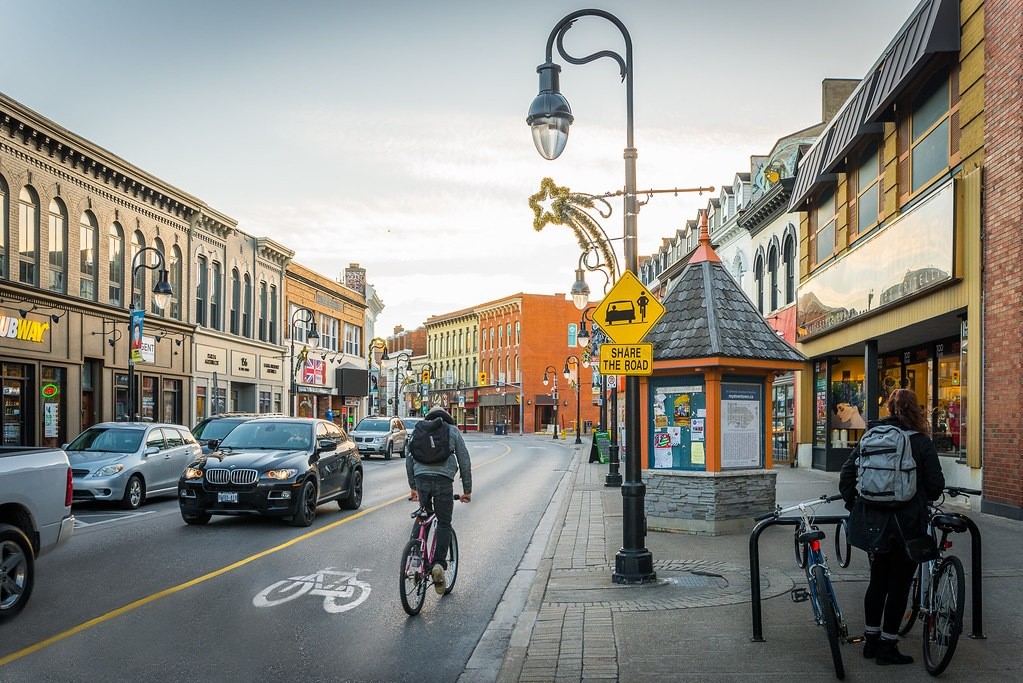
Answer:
[425,406,454,425]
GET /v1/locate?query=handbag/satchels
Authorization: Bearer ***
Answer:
[905,535,941,562]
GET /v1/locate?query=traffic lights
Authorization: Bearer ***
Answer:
[422,370,430,384]
[478,373,487,386]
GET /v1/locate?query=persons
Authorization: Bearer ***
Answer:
[285,427,311,445]
[836,384,945,667]
[300,396,313,417]
[131,322,142,349]
[406,403,473,595]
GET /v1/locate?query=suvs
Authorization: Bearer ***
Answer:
[349,416,408,460]
[176,417,364,527]
[191,411,290,457]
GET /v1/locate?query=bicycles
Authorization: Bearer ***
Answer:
[753,494,866,682]
[399,495,468,616]
[899,485,984,679]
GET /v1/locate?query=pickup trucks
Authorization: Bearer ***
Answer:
[0,442,76,623]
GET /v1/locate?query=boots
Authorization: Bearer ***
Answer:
[877,638,916,667]
[863,631,884,658]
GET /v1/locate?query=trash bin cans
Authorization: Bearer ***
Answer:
[494,424,503,435]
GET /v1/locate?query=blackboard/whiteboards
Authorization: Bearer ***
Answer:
[589,431,611,464]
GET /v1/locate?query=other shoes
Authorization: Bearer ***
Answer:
[407,556,422,577]
[433,565,447,594]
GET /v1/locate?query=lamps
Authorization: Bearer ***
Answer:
[92,329,122,347]
[161,329,187,346]
[34,303,66,324]
[0,298,35,318]
[306,348,345,364]
[142,326,167,343]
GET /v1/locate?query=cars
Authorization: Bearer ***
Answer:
[64,418,202,508]
[400,418,424,437]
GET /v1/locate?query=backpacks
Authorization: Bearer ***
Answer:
[410,418,453,464]
[856,427,925,501]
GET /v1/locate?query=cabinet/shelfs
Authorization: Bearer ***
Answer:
[3,393,22,447]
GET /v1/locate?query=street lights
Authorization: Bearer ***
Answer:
[496,381,524,436]
[395,352,413,417]
[456,380,467,433]
[129,246,172,422]
[577,307,607,433]
[526,9,656,582]
[563,355,582,444]
[421,363,435,418]
[369,337,390,416]
[289,307,321,415]
[571,247,622,488]
[542,366,558,439]
